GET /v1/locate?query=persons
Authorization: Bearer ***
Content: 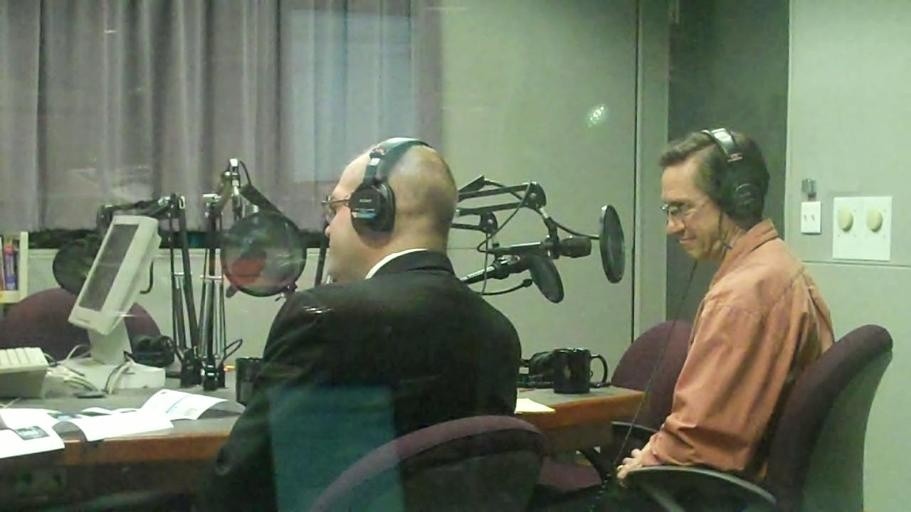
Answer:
[209,133,524,512]
[544,127,838,512]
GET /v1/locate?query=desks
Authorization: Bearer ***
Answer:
[1,375,647,470]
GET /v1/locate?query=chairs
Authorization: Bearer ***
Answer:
[312,416,546,512]
[623,324,893,511]
[577,320,691,473]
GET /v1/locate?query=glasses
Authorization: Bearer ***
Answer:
[661,194,712,220]
[320,193,353,217]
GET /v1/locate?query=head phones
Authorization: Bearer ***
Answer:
[350,137,432,239]
[699,126,764,219]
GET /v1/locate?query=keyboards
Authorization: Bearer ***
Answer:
[0,346,49,374]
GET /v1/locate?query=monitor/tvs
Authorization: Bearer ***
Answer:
[67,216,166,389]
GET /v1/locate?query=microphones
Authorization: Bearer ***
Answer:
[459,254,528,285]
[225,250,266,300]
[499,237,591,260]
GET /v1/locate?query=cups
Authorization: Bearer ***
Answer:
[551,347,609,395]
